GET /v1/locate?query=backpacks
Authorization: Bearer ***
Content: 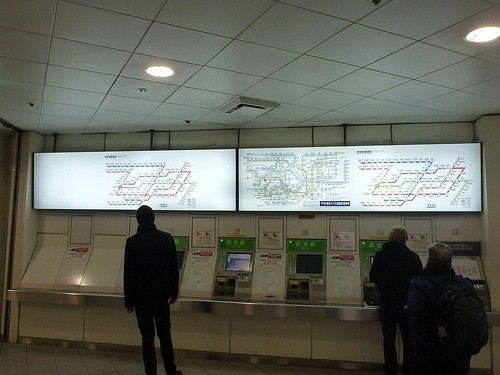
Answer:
[416,273,489,355]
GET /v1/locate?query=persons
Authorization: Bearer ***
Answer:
[369,224,424,375]
[407,241,476,375]
[124,205,183,375]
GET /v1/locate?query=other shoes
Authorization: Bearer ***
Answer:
[173,370,183,375]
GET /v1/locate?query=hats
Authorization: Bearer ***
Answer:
[136,205,155,227]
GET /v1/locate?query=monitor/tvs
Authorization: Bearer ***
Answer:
[451,255,481,279]
[225,253,251,271]
[296,254,322,274]
[176,250,184,269]
